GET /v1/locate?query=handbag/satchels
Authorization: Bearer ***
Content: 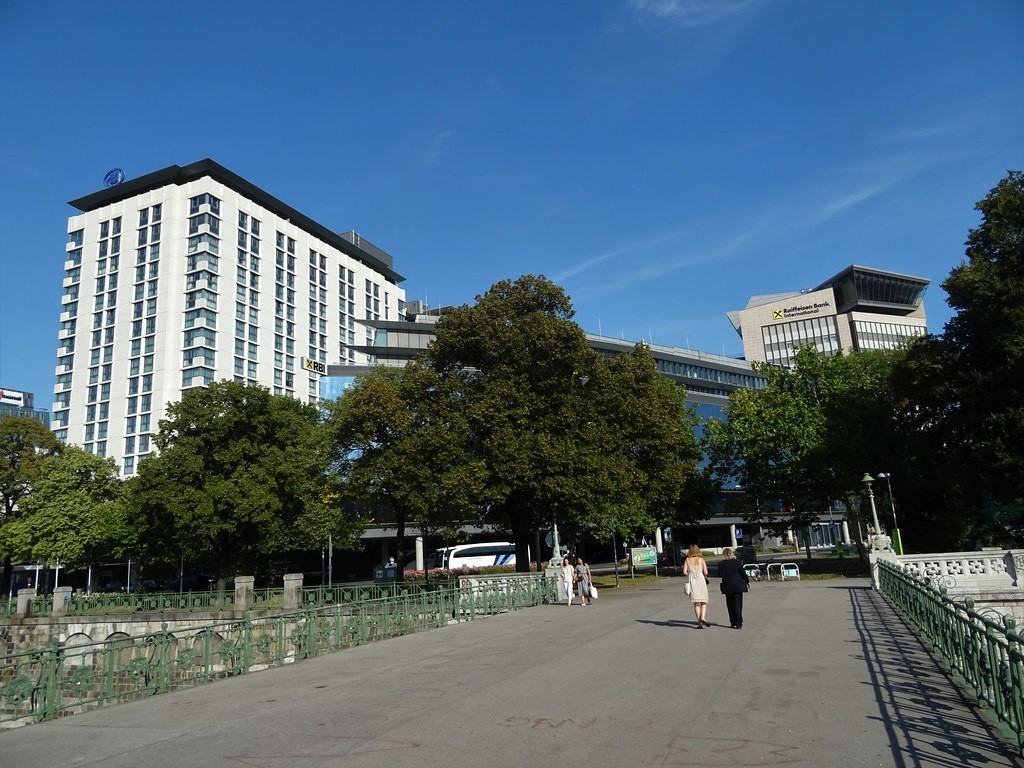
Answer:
[705,578,709,585]
[589,584,598,599]
[684,582,690,594]
[577,575,584,582]
[720,583,725,595]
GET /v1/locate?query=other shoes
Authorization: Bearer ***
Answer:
[732,626,741,629]
[701,620,710,627]
[581,604,585,606]
[697,627,703,629]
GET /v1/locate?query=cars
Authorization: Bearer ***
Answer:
[657,550,687,567]
[207,573,238,590]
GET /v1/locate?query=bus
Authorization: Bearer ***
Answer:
[435,542,531,570]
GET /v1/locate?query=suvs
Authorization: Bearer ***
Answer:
[127,580,167,593]
[100,581,133,593]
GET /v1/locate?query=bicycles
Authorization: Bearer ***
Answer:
[750,559,779,582]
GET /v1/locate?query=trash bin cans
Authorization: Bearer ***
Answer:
[740,546,756,565]
[372,563,397,583]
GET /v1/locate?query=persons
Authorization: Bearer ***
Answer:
[717,548,751,629]
[682,544,710,629]
[560,557,578,606]
[573,557,592,606]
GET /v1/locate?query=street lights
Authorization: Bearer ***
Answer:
[860,472,883,550]
[878,472,903,555]
